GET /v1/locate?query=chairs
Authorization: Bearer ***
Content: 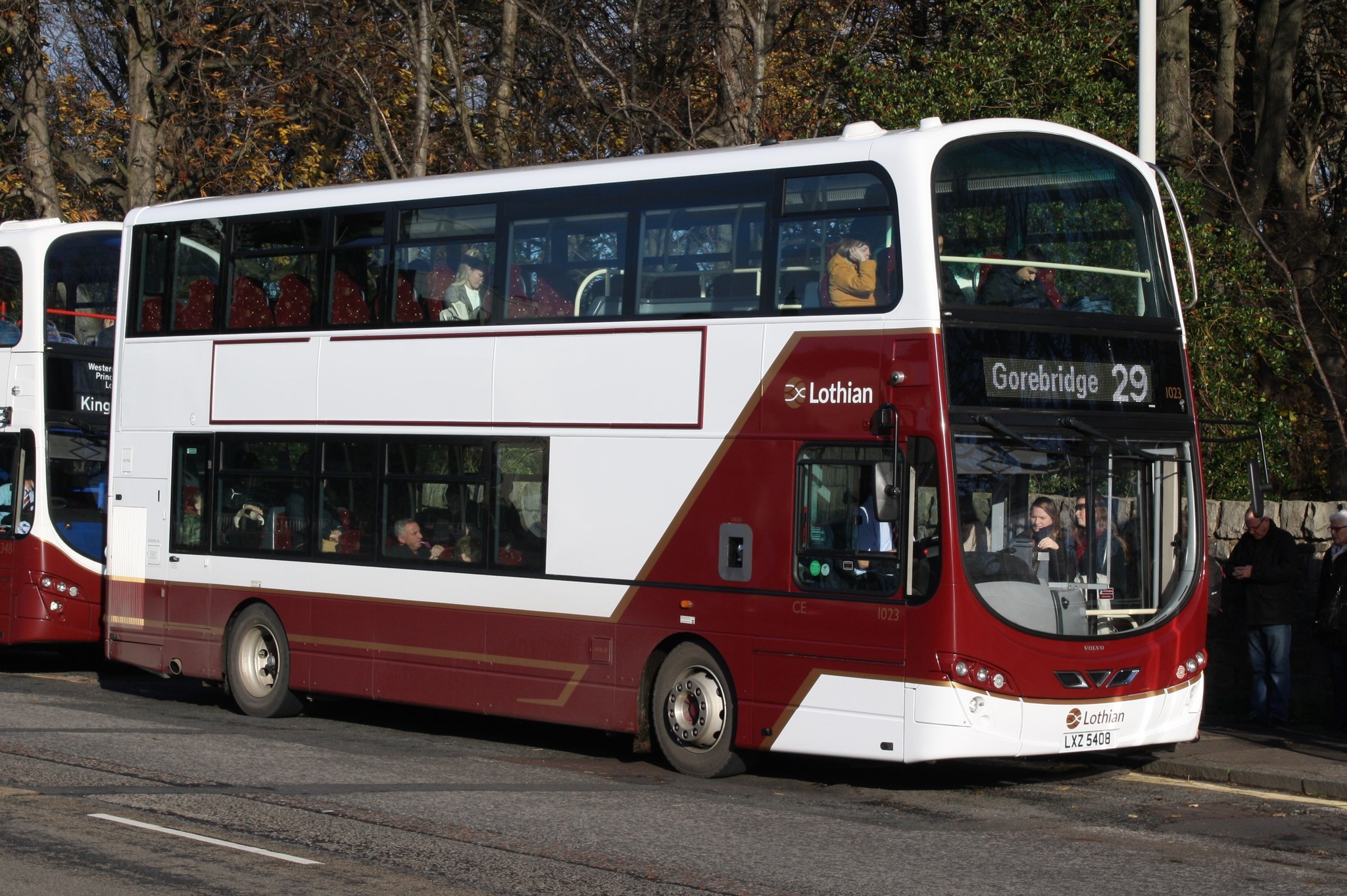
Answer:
[817,242,838,309]
[262,506,292,551]
[142,298,185,332]
[337,530,361,556]
[498,550,523,567]
[533,271,578,318]
[439,547,458,562]
[229,276,275,328]
[183,279,216,330]
[428,263,456,321]
[332,270,371,323]
[275,273,316,327]
[0,314,21,344]
[972,253,1004,301]
[183,485,199,511]
[375,274,423,323]
[876,246,895,297]
[338,509,350,531]
[483,262,534,318]
[46,318,115,348]
[426,271,434,291]
[1035,267,1064,311]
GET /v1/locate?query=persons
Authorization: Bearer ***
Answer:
[843,493,915,576]
[1174,511,1223,696]
[1075,490,1128,631]
[936,232,968,304]
[977,244,1054,310]
[441,248,487,326]
[960,509,990,554]
[1227,506,1301,737]
[177,469,529,569]
[1014,496,1077,583]
[0,463,36,534]
[827,231,876,308]
[1321,510,1346,691]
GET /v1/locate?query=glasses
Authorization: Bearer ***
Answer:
[1327,526,1347,533]
[1243,518,1265,531]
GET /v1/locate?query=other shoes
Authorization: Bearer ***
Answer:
[1263,716,1285,727]
[1239,713,1262,724]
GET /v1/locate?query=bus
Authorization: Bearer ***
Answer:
[0,216,274,645]
[100,116,1276,779]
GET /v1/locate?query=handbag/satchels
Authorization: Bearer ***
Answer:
[1325,592,1347,632]
